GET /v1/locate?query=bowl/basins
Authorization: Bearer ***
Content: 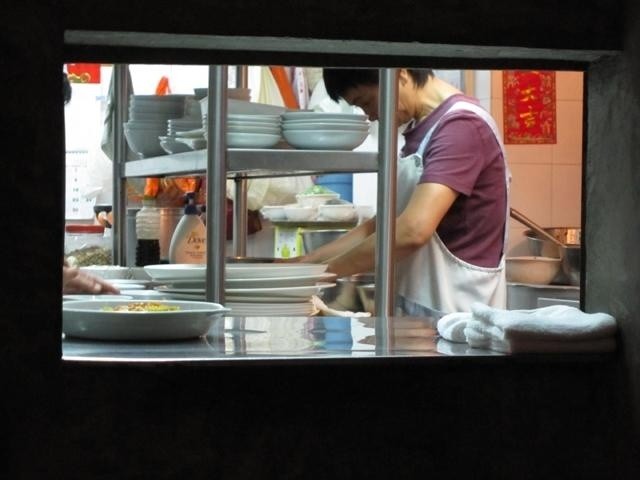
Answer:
[521,226,582,287]
[506,255,563,285]
[319,274,377,315]
[260,194,373,227]
[123,86,252,158]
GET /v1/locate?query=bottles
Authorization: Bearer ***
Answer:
[64,223,113,267]
[136,195,161,267]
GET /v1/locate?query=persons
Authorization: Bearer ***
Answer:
[63,256,121,296]
[272,64,510,315]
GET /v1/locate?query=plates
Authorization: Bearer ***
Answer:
[62,299,231,340]
[63,260,164,300]
[161,111,373,155]
[145,261,338,316]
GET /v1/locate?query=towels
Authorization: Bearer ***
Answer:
[438,305,619,354]
[101,64,145,195]
